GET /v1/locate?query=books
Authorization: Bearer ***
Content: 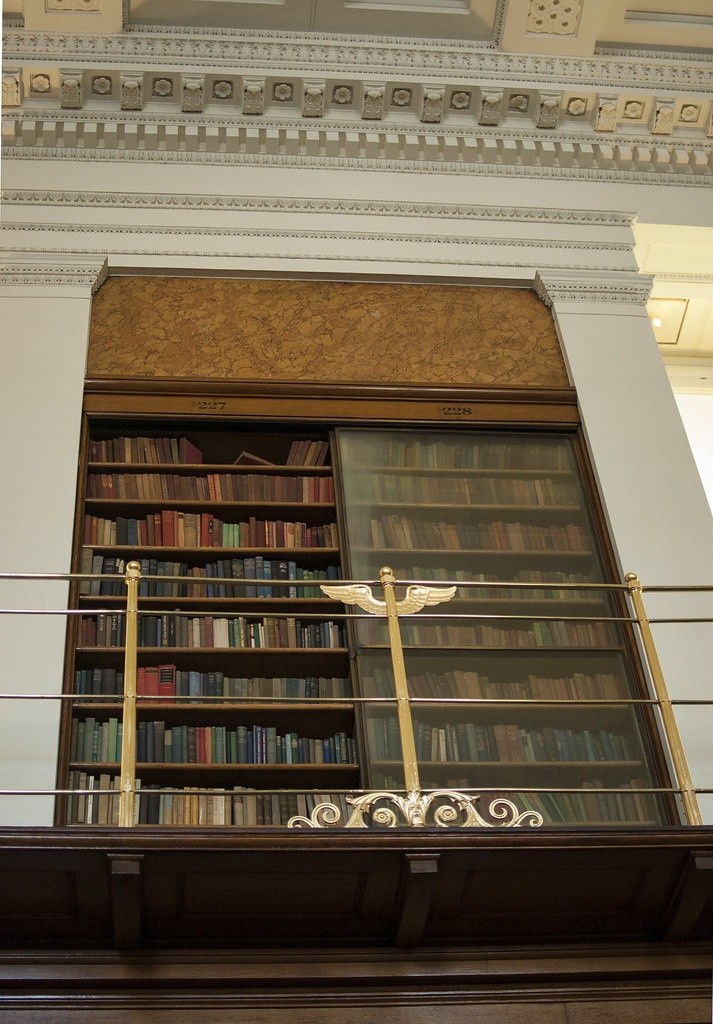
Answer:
[89,427,331,466]
[72,607,349,651]
[364,660,632,709]
[349,467,580,509]
[66,768,368,826]
[360,711,635,766]
[343,430,573,474]
[70,661,358,708]
[76,547,343,598]
[372,769,652,821]
[85,471,338,505]
[356,557,604,607]
[356,610,613,650]
[77,509,340,551]
[349,509,590,554]
[68,713,360,766]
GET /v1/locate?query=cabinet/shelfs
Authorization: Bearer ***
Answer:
[52,392,681,830]
